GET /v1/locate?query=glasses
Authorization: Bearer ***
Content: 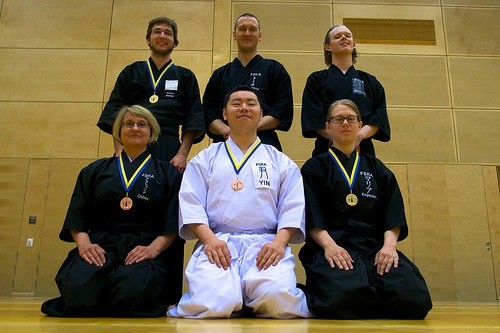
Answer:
[121,121,150,128]
[328,115,360,124]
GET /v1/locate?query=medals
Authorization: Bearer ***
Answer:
[149,95,159,103]
[231,179,243,191]
[120,197,133,210]
[346,194,358,206]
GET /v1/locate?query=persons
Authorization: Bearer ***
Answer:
[97,16,206,173]
[203,13,293,152]
[296,99,433,320]
[40,105,186,319]
[166,85,313,319]
[301,25,390,158]
